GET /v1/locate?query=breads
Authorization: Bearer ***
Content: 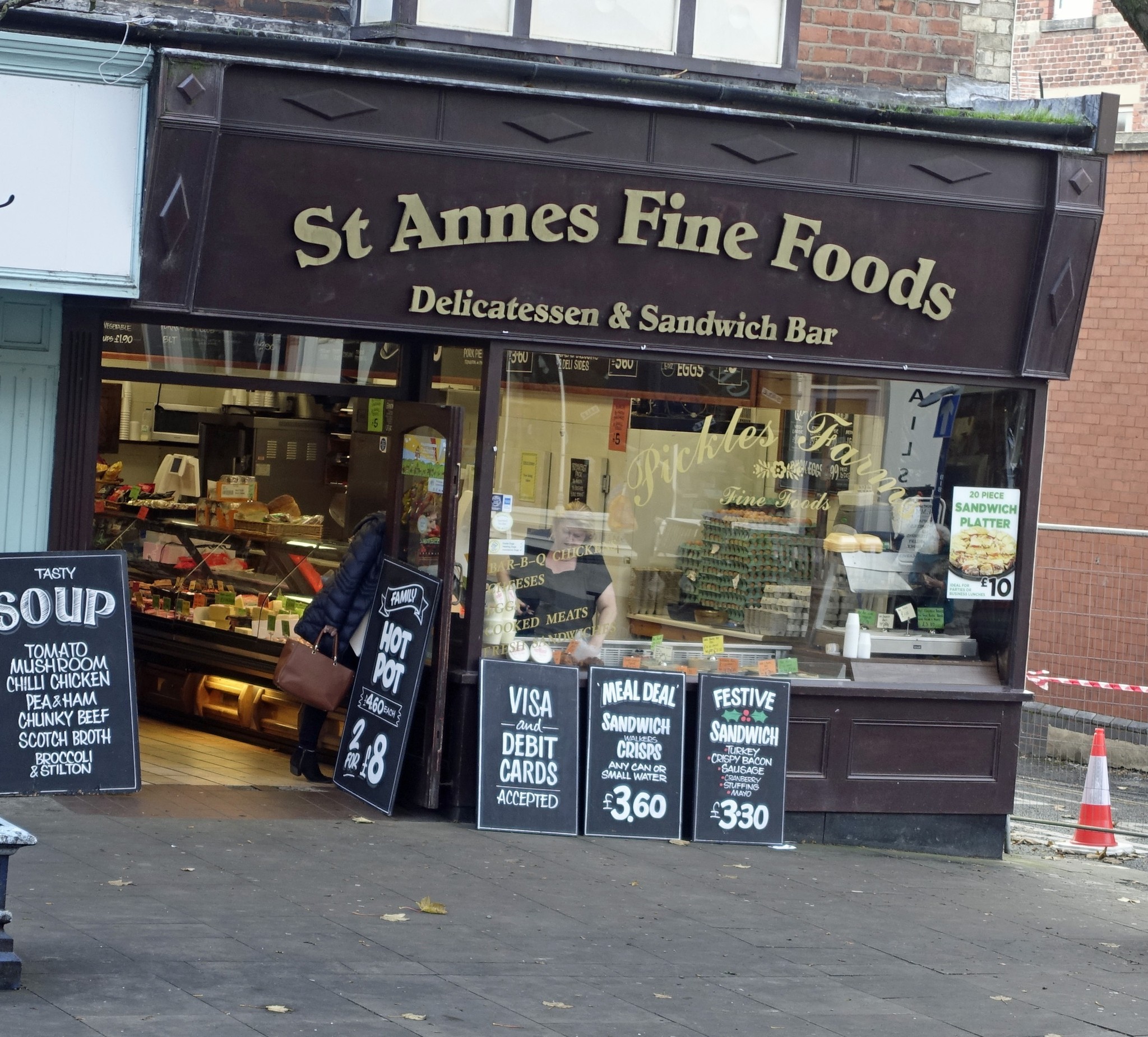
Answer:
[267,493,302,517]
[96,459,122,481]
[237,500,270,522]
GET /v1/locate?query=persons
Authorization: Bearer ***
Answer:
[291,481,441,784]
[909,523,954,635]
[496,500,618,660]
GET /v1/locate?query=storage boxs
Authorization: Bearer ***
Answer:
[106,500,325,536]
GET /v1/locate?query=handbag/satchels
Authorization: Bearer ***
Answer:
[273,628,353,714]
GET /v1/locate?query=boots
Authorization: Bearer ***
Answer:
[289,746,333,784]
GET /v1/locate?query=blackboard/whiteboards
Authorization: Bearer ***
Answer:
[475,657,580,837]
[583,665,687,842]
[332,556,444,817]
[0,549,143,796]
[692,671,792,847]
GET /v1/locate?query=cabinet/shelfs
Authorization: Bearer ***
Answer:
[88,511,389,761]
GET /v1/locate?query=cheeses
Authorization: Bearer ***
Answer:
[200,594,282,636]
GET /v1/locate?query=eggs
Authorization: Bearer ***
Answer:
[686,509,813,609]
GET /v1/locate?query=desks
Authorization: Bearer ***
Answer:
[626,612,805,660]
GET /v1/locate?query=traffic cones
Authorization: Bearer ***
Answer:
[1070,726,1118,848]
